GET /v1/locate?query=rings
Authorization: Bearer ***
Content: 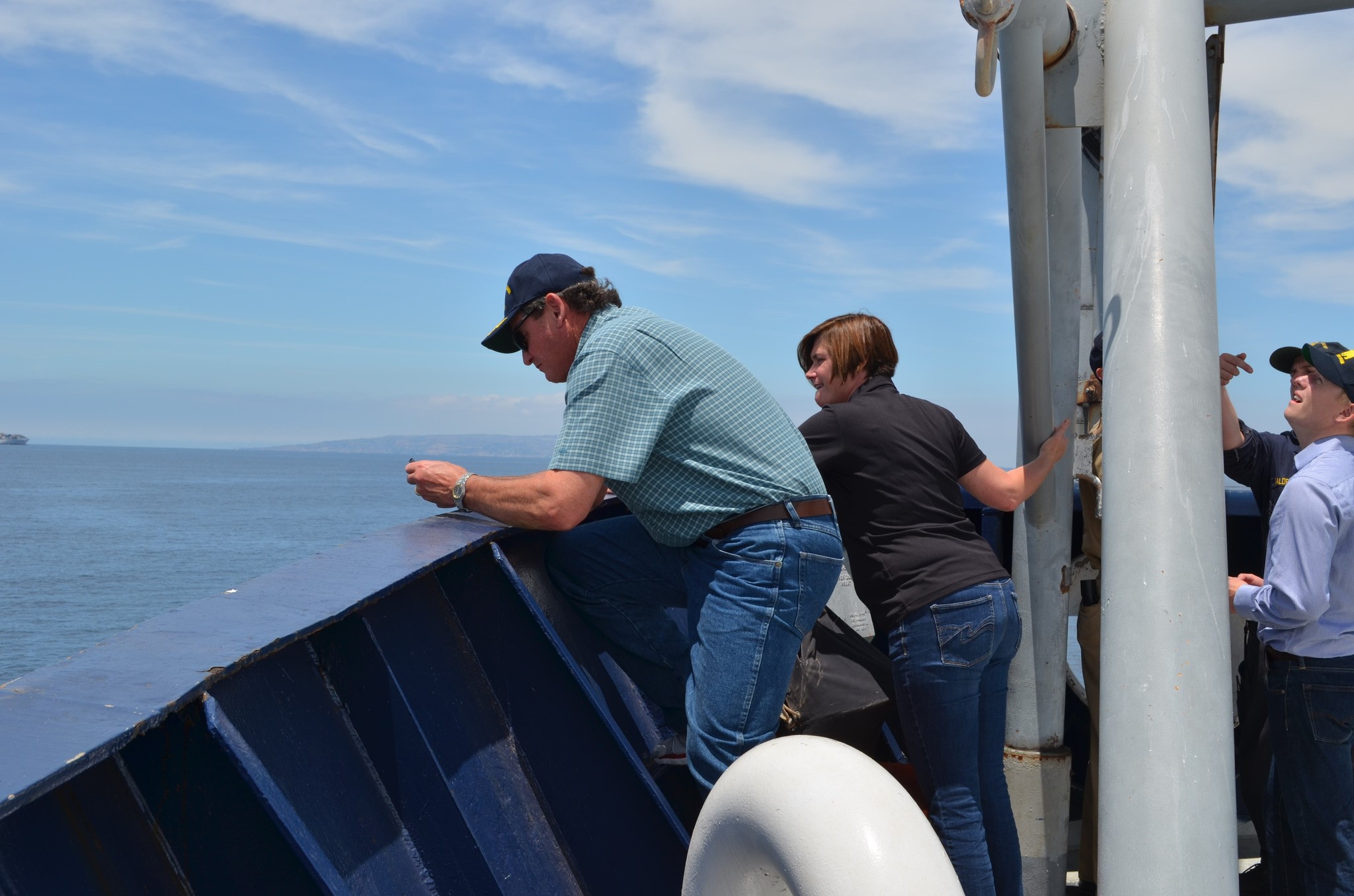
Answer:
[415,486,421,497]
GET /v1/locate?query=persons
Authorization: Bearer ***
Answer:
[406,251,847,797]
[795,310,1073,896]
[1077,329,1102,896]
[1219,340,1354,896]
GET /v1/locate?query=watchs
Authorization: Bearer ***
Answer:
[451,472,478,513]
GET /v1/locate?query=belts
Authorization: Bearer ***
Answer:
[695,498,833,548]
[1266,646,1313,662]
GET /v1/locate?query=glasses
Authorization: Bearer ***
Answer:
[509,302,542,351]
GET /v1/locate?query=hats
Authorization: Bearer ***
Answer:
[1269,341,1351,375]
[1301,343,1354,404]
[480,253,597,355]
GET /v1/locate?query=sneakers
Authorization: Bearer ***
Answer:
[651,733,688,765]
